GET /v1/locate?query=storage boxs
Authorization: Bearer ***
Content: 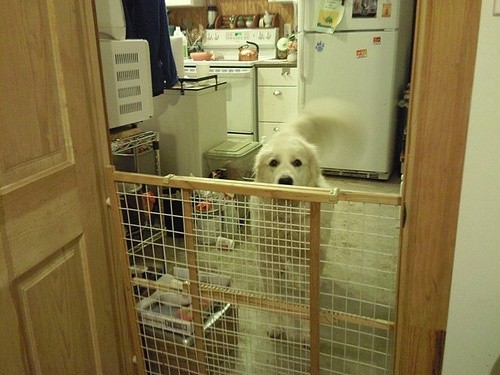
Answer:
[204,139,263,180]
[141,307,240,375]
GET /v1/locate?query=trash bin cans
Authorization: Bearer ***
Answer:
[203,140,262,227]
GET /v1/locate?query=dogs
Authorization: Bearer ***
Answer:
[249,112,351,344]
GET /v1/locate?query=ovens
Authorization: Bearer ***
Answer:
[183,61,258,143]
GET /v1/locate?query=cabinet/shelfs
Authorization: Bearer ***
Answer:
[110,131,167,257]
[257,68,298,144]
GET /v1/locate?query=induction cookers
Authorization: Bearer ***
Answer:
[185,28,278,61]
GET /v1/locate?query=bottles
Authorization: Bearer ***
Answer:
[207,6,217,28]
[169,24,205,60]
[229,15,253,29]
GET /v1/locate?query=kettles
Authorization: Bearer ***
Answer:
[238,42,259,61]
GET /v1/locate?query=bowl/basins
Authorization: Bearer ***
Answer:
[190,53,212,60]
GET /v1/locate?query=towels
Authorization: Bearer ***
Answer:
[121,0,180,98]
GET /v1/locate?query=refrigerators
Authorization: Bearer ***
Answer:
[294,0,415,181]
